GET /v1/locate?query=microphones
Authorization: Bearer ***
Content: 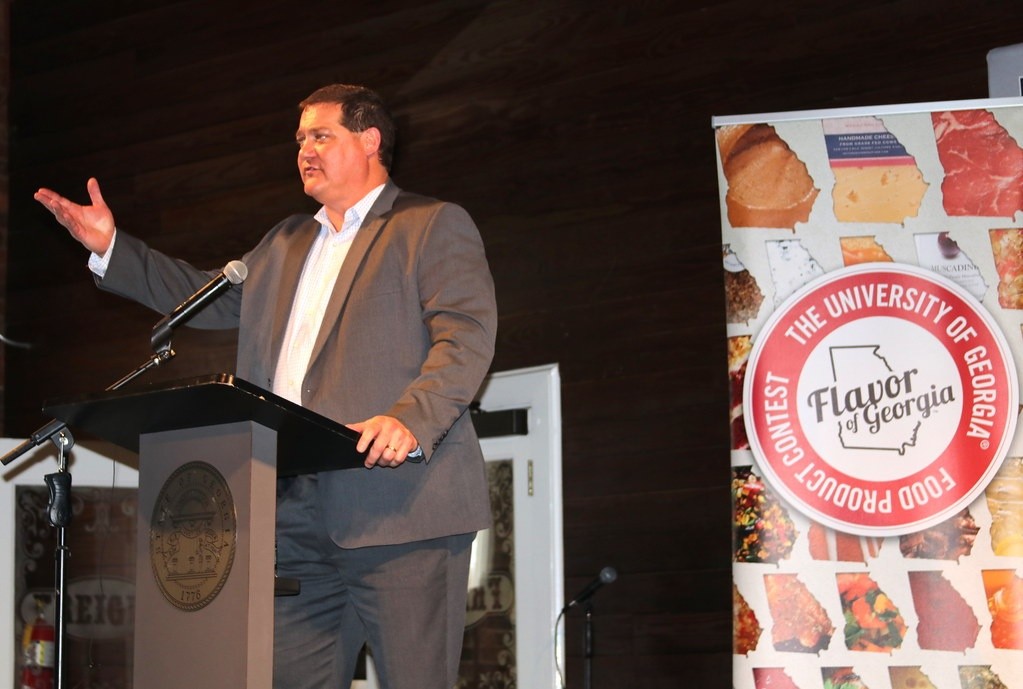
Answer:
[564,566,617,613]
[151,260,248,350]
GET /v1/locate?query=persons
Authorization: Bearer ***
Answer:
[35,85,498,689]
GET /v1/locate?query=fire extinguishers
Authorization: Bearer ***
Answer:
[20,593,56,689]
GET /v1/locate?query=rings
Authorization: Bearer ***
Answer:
[386,445,396,452]
